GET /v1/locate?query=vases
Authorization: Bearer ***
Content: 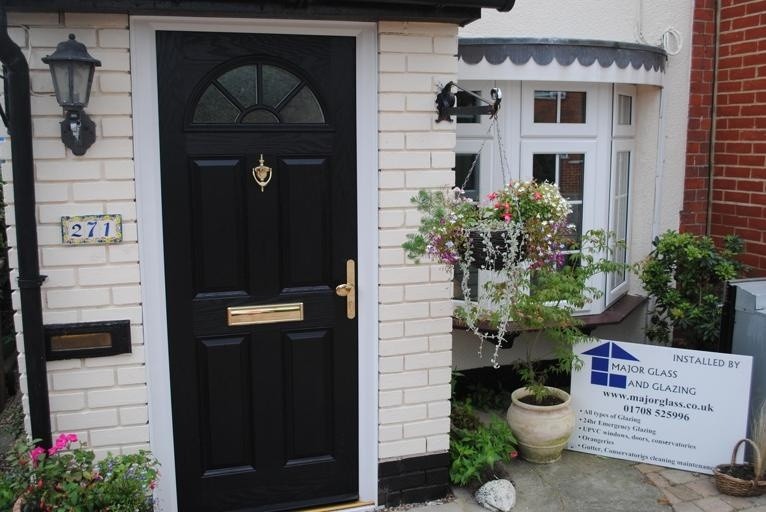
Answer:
[8,482,71,510]
[454,225,529,271]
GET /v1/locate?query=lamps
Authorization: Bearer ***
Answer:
[43,35,101,154]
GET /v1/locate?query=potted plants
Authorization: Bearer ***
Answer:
[506,227,621,465]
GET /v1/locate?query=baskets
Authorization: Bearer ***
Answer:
[713,463,766,497]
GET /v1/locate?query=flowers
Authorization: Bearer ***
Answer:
[2,433,162,511]
[417,170,578,372]
